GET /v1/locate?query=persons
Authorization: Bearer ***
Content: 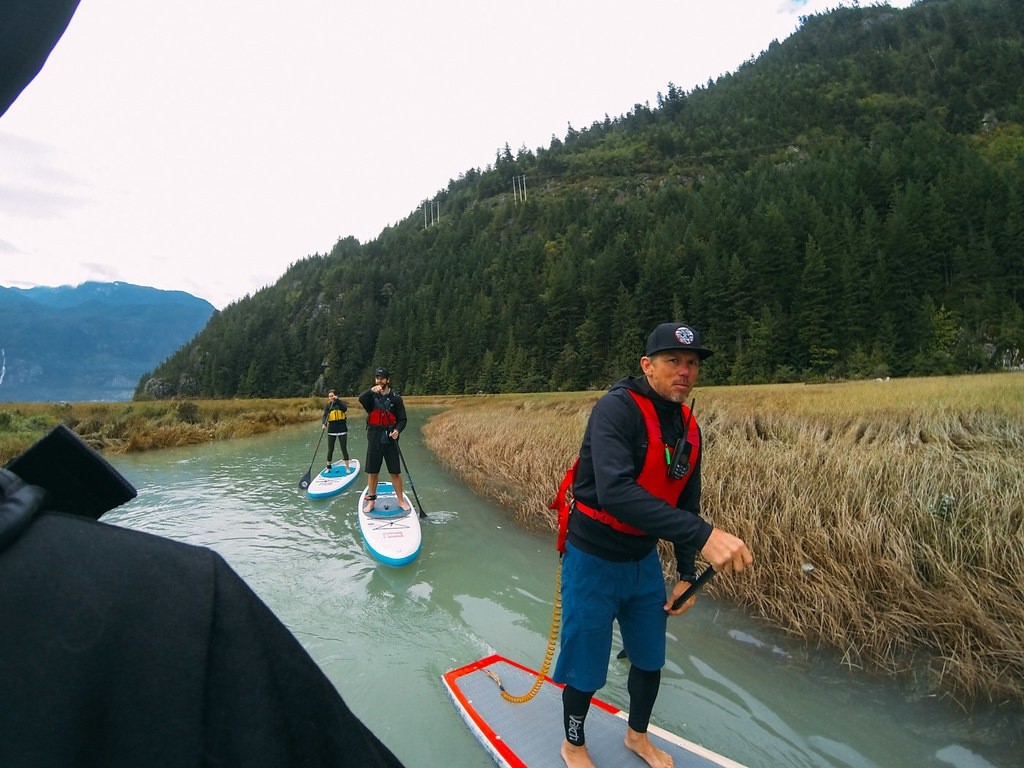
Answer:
[359,368,411,513]
[0,425,418,767]
[322,389,352,474]
[558,320,752,768]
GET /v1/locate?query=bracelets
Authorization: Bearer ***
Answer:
[323,425,326,426]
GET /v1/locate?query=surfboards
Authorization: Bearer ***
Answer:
[358,482,422,567]
[306,459,361,500]
[440,654,749,768]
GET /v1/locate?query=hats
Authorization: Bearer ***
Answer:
[375,368,389,380]
[646,322,714,362]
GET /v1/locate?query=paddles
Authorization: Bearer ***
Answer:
[617,566,717,659]
[379,392,428,519]
[299,398,335,489]
